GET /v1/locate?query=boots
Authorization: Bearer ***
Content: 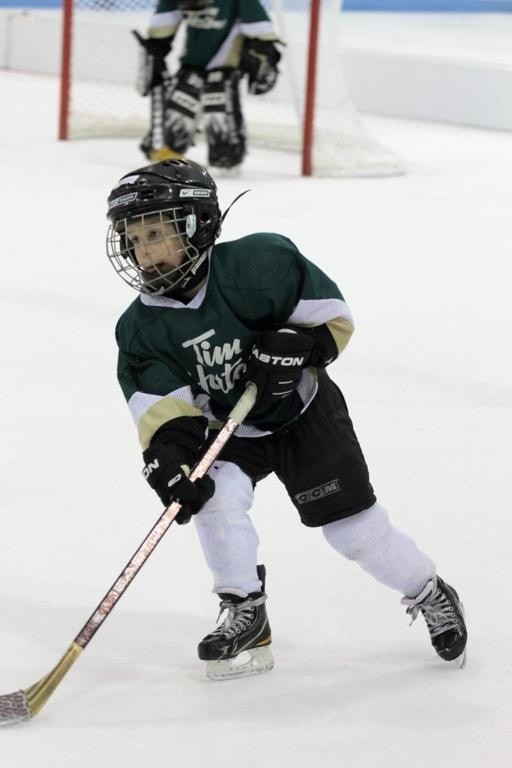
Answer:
[402,576,466,662]
[199,566,271,661]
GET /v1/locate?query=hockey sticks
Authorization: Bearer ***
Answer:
[0,381,258,726]
[149,58,186,164]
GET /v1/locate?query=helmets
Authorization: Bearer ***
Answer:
[106,158,221,299]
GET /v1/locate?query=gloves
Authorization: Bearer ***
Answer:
[236,331,313,419]
[144,417,215,528]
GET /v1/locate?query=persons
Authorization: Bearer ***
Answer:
[105,158,468,661]
[132,0,287,169]
